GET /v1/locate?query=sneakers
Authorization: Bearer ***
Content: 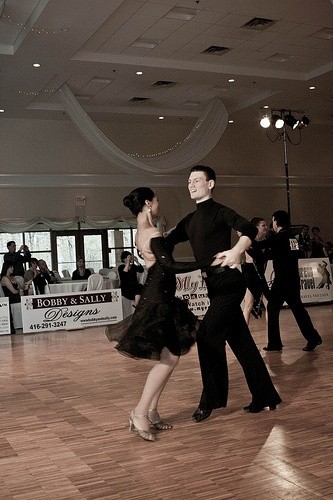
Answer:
[263,344,284,352]
[302,338,323,351]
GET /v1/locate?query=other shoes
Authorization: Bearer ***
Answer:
[132,304,135,309]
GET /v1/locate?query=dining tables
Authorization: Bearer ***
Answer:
[44,283,88,295]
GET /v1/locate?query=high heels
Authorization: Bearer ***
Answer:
[244,396,282,413]
[148,408,174,430]
[192,395,229,422]
[128,409,156,442]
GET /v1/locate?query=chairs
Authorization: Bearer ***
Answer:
[0,267,122,328]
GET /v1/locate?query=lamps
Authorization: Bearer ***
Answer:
[269,110,285,129]
[297,116,311,130]
[284,109,300,129]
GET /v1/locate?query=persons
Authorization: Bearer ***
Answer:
[104,187,227,442]
[72,258,92,280]
[118,251,144,308]
[243,209,333,352]
[138,165,282,422]
[0,241,58,303]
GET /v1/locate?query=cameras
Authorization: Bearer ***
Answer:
[131,256,135,259]
[24,245,27,248]
